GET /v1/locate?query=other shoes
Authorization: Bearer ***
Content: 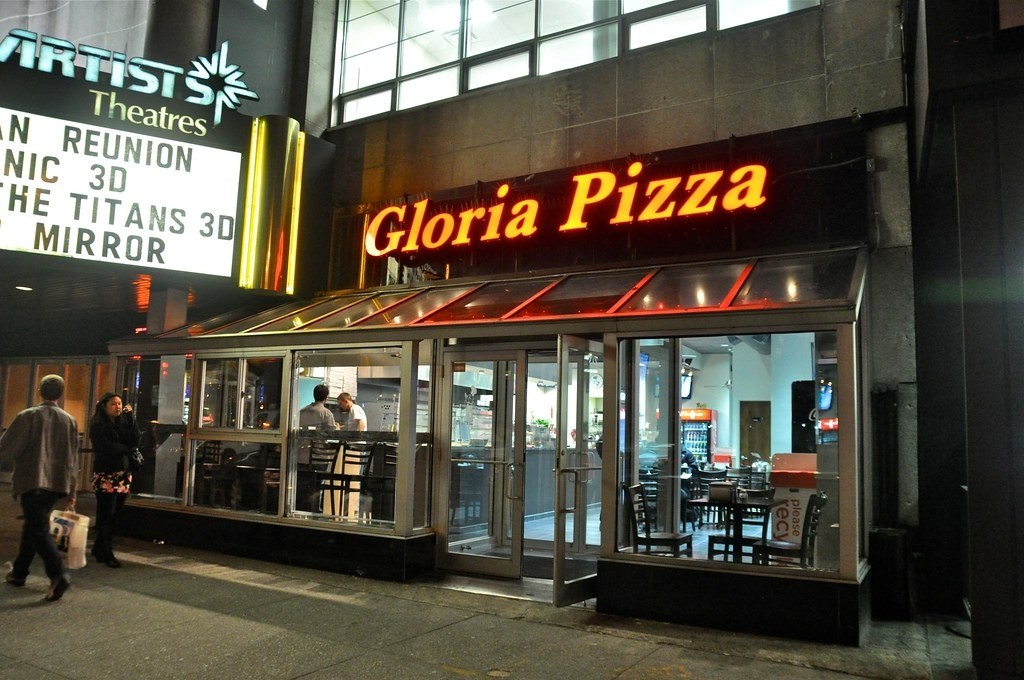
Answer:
[46,576,69,600]
[6,573,25,585]
[106,556,120,568]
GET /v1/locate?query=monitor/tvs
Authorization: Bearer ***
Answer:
[681,375,692,399]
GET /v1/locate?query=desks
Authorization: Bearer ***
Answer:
[689,495,789,564]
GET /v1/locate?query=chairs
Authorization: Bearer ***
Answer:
[621,453,827,570]
[174,437,399,523]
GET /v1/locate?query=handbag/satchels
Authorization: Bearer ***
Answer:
[131,447,144,473]
[49,502,90,570]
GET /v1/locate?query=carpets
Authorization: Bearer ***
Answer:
[479,549,598,582]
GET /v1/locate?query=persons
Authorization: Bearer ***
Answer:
[680,444,698,521]
[0,374,79,603]
[299,385,340,513]
[639,442,658,473]
[337,393,367,472]
[88,392,141,568]
